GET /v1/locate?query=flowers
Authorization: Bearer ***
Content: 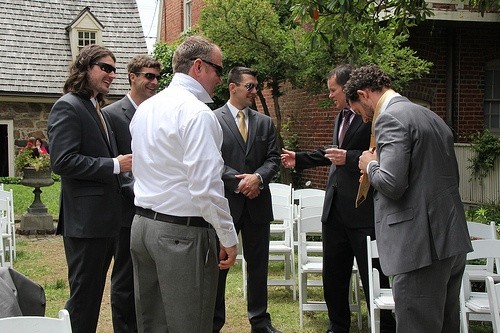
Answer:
[15,148,51,174]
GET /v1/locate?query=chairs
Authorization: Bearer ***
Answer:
[457,220,500,333]
[0,183,73,333]
[239,177,395,333]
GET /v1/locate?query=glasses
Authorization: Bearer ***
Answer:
[133,71,161,81]
[91,61,116,74]
[232,81,258,91]
[189,57,223,76]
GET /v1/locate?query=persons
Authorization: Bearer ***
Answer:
[48,45,131,333]
[17,142,33,155]
[129,35,239,333]
[343,63,474,333]
[101,54,160,333]
[211,67,284,333]
[33,138,48,154]
[280,65,396,333]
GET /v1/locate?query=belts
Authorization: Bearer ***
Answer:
[135,206,210,227]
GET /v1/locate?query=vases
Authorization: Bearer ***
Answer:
[22,167,55,211]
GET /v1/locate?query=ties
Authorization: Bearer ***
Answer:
[356,88,393,208]
[338,109,353,149]
[95,102,109,144]
[237,110,248,143]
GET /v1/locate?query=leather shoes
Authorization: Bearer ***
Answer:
[253,325,281,333]
